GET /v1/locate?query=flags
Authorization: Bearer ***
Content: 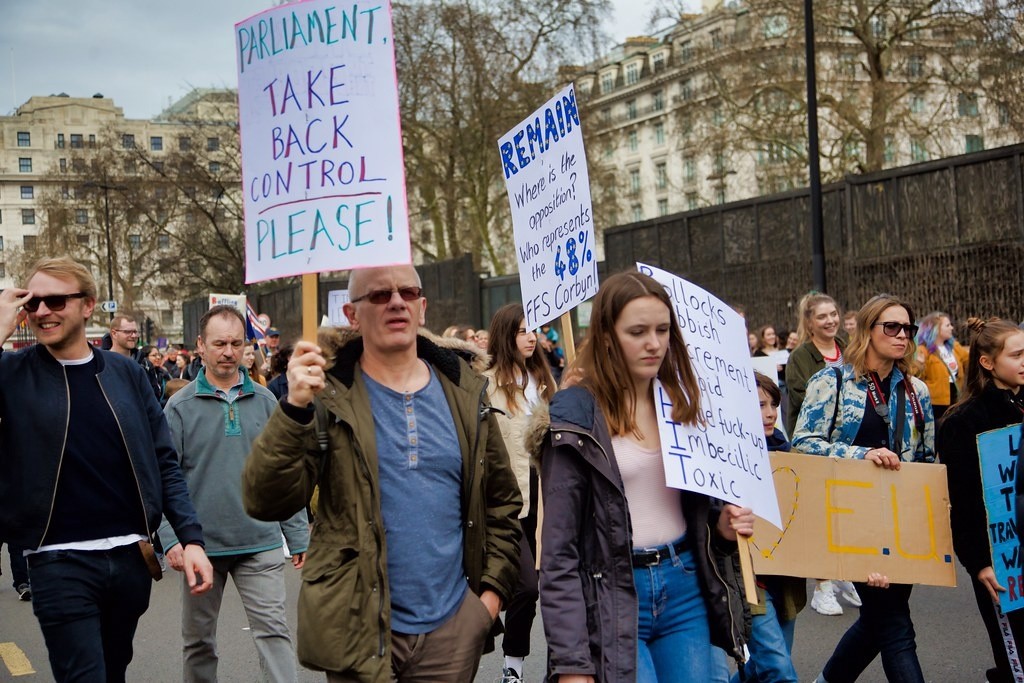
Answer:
[240,295,266,350]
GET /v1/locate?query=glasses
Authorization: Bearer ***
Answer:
[468,335,478,339]
[115,329,138,336]
[351,286,422,305]
[873,321,918,338]
[21,292,88,312]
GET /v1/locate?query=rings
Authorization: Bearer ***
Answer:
[877,452,880,456]
[308,366,311,374]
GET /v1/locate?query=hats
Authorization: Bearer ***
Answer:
[265,327,281,336]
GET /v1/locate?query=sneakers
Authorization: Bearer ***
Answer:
[832,578,861,606]
[811,581,844,615]
[19,584,31,600]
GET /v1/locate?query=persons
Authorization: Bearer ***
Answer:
[0,259,1024,683]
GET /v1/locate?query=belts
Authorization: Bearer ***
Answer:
[634,538,690,567]
